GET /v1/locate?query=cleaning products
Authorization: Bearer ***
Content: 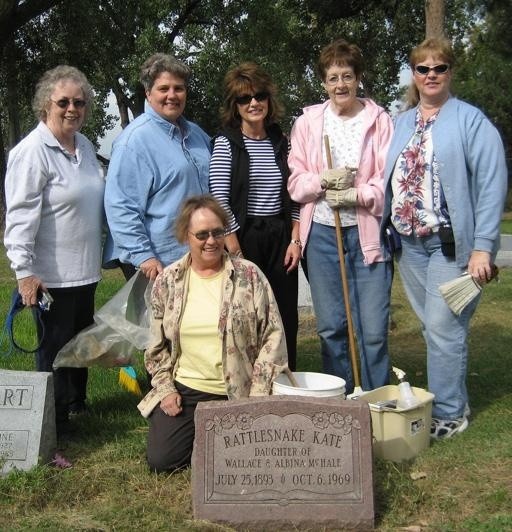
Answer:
[389,366,418,410]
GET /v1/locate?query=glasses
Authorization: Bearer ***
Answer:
[326,74,357,85]
[415,63,450,75]
[48,98,86,108]
[189,229,226,239]
[236,92,266,104]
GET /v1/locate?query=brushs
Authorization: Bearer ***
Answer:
[440,263,499,317]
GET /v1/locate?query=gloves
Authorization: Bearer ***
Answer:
[320,167,357,190]
[325,187,358,209]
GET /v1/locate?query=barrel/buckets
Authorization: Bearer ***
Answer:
[272,371,348,401]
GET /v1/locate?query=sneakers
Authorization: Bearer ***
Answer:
[57,402,91,437]
[430,402,471,441]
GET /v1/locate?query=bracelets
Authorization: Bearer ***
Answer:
[290,238,303,248]
[234,248,242,258]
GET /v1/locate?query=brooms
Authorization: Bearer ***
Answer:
[117,365,142,398]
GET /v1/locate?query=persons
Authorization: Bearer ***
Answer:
[104,55,215,389]
[3,66,106,443]
[136,198,289,476]
[209,62,305,373]
[287,37,395,399]
[377,38,509,443]
